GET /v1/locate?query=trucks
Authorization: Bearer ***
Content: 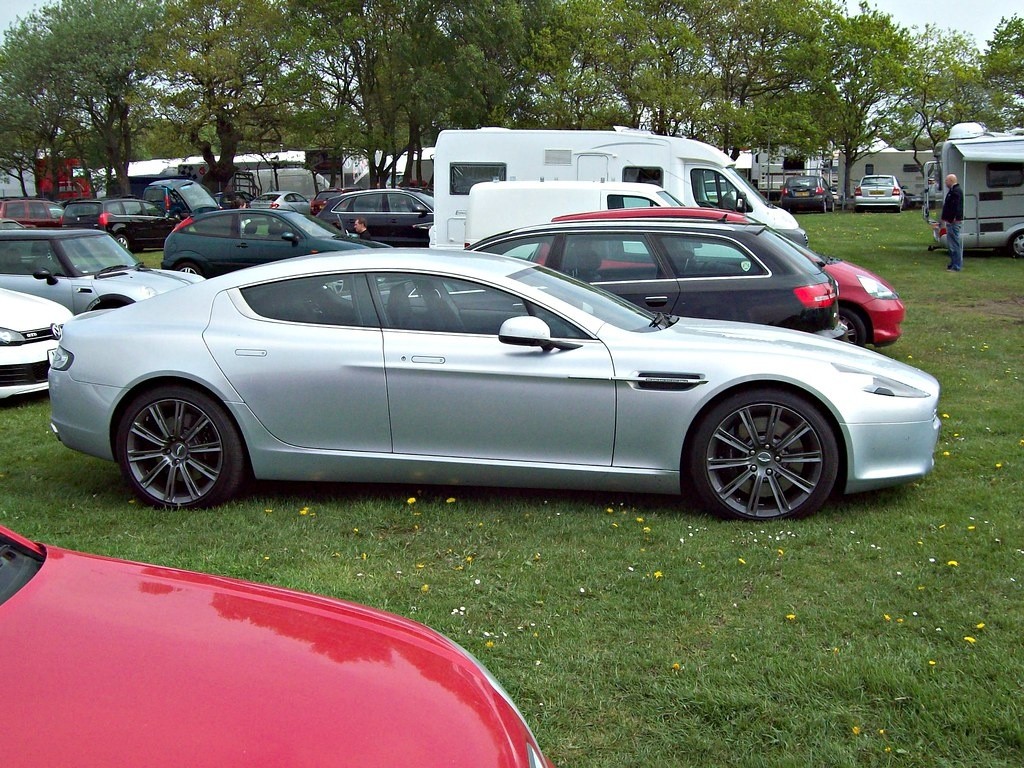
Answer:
[922,122,1024,258]
[429,125,809,250]
[0,145,434,201]
[837,151,942,206]
[832,160,838,190]
[751,141,832,199]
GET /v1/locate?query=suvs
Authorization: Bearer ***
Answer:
[316,191,434,247]
[61,197,184,253]
[340,218,850,343]
[311,186,368,216]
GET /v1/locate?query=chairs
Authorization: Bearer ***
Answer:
[30,244,59,273]
[264,282,467,334]
[575,252,603,284]
[2,249,30,275]
[244,222,260,240]
[266,222,284,240]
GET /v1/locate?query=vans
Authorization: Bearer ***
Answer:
[466,181,747,261]
[143,180,215,220]
[224,168,330,201]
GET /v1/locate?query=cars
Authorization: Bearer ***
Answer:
[0,217,39,252]
[0,197,65,229]
[0,288,75,401]
[398,179,428,189]
[386,173,406,188]
[247,190,312,224]
[149,180,394,291]
[0,526,556,768]
[0,228,207,314]
[854,174,905,212]
[528,208,905,348]
[780,176,835,214]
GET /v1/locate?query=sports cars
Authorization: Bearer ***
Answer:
[46,244,941,522]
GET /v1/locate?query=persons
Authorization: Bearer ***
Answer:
[332,221,348,236]
[941,174,965,272]
[353,217,371,240]
[231,199,251,229]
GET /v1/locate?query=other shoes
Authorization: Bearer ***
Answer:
[947,268,956,272]
